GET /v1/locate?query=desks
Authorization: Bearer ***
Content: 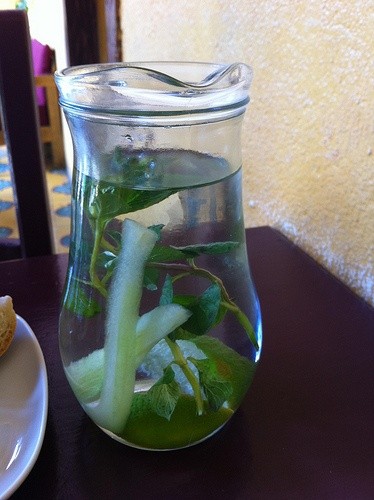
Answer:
[0,226,374,500]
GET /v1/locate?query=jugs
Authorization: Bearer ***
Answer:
[53,60,262,452]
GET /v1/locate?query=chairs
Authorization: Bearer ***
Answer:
[0,8,54,252]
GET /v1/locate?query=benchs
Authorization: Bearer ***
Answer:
[0,39,64,169]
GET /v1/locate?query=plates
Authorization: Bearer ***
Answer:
[0,314,49,500]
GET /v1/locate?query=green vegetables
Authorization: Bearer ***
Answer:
[63,145,260,423]
